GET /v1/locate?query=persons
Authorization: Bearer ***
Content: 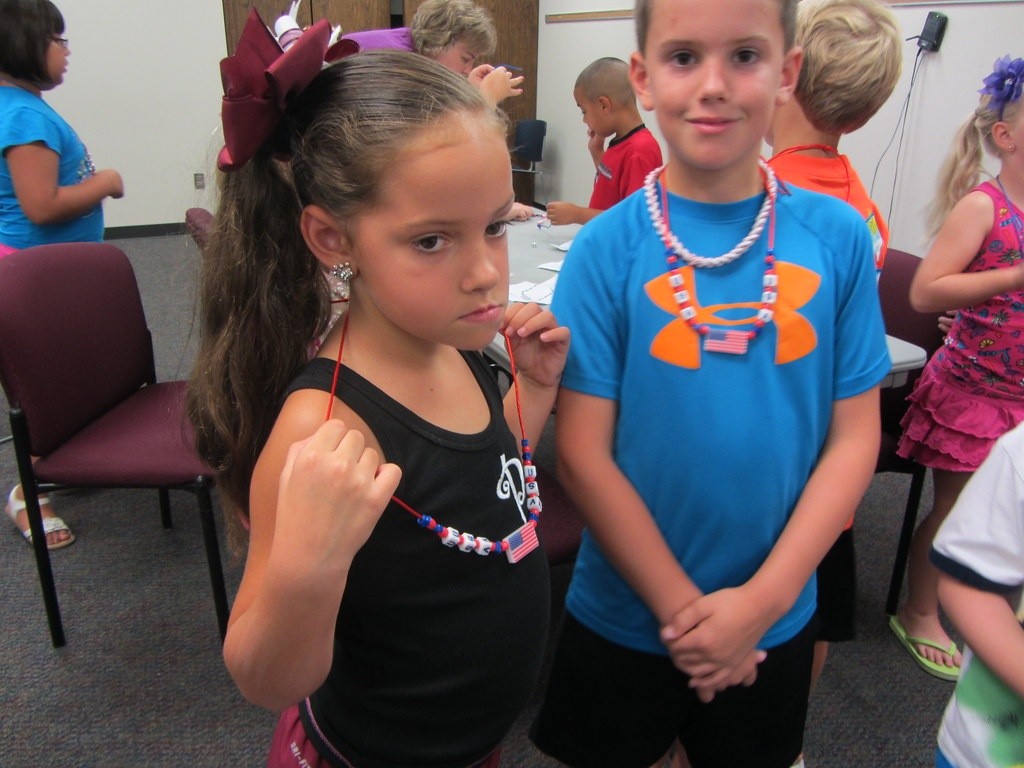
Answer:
[888,56,1024,679]
[0,0,124,550]
[528,0,892,768]
[767,1,901,710]
[927,423,1024,768]
[339,0,524,110]
[183,11,571,768]
[546,57,664,223]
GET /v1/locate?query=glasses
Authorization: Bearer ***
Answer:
[49,37,69,49]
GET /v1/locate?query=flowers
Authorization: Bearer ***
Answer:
[978,55,1023,121]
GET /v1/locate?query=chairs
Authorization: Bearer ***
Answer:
[1,241,231,651]
[874,247,955,616]
[184,206,215,250]
[510,118,548,204]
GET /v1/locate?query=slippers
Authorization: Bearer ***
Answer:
[889,614,961,682]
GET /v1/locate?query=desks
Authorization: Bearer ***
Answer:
[483,205,928,415]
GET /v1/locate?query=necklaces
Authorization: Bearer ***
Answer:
[322,312,543,563]
[644,159,778,268]
[657,166,777,354]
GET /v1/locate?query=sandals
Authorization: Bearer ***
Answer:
[6,482,75,550]
